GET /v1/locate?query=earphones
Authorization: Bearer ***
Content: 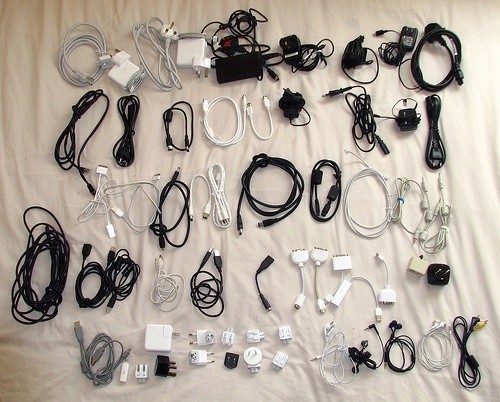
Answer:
[389,320,397,329]
[394,324,402,330]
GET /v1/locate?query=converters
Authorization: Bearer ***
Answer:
[215,51,262,84]
